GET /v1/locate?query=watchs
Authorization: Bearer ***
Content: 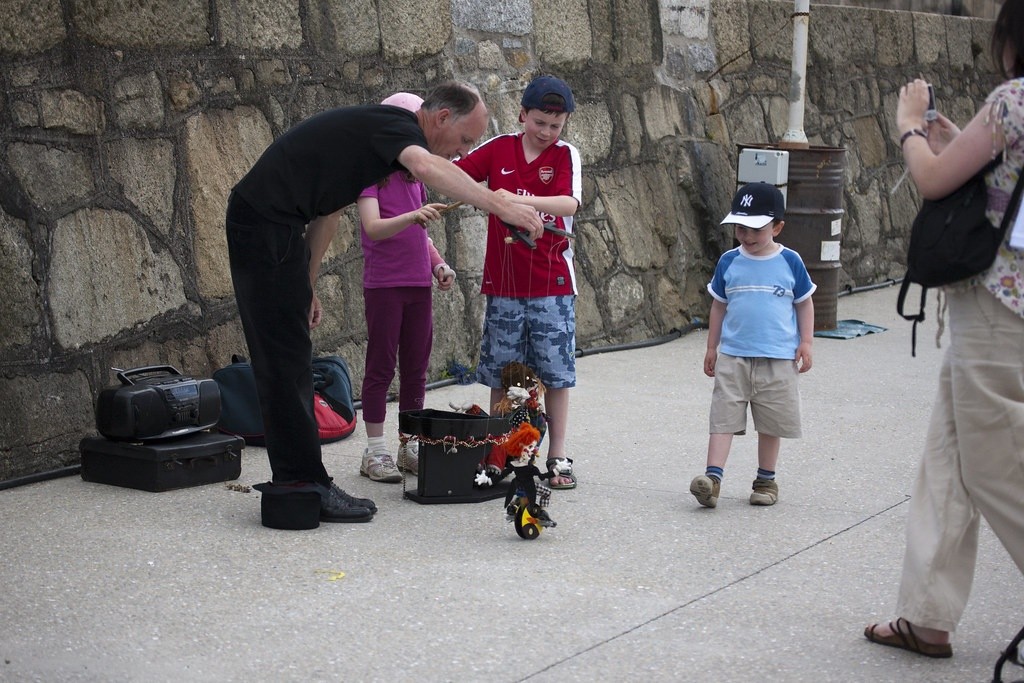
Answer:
[901,129,928,147]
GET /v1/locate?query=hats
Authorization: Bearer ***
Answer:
[718,182,784,227]
[380,92,424,113]
[518,76,575,123]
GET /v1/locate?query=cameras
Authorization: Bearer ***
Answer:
[925,84,937,121]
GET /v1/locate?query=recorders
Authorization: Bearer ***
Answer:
[95,365,222,442]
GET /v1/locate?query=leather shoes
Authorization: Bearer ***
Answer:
[317,490,373,524]
[330,482,378,515]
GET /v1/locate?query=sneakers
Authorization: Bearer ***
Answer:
[360,448,401,483]
[750,479,778,506]
[397,443,420,474]
[690,476,722,509]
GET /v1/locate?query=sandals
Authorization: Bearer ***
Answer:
[546,457,577,489]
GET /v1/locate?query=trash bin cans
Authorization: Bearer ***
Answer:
[734,142,846,332]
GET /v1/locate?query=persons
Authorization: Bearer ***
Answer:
[450,76,584,490]
[357,93,456,483]
[864,0,1024,659]
[225,80,545,523]
[690,182,817,508]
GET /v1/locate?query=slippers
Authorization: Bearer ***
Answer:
[866,619,955,659]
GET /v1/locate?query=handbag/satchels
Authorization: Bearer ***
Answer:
[906,155,1024,289]
[208,353,356,444]
